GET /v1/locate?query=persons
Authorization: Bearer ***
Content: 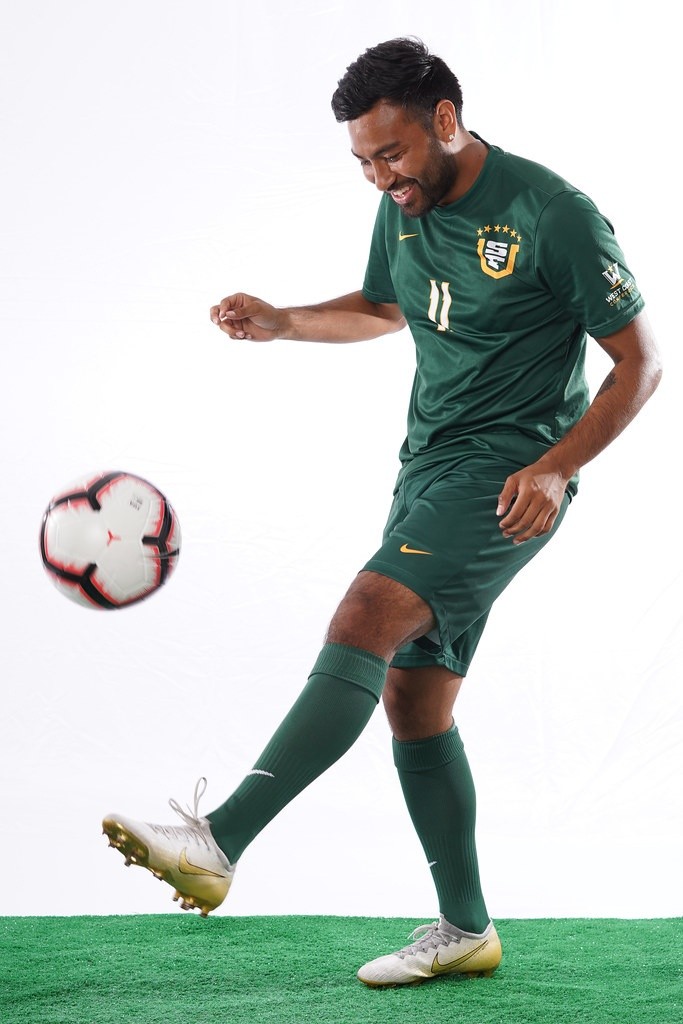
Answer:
[101,36,663,990]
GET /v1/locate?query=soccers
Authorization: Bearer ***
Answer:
[41,471,181,610]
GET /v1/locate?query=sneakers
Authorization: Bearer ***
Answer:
[353,915,504,984]
[97,811,235,919]
